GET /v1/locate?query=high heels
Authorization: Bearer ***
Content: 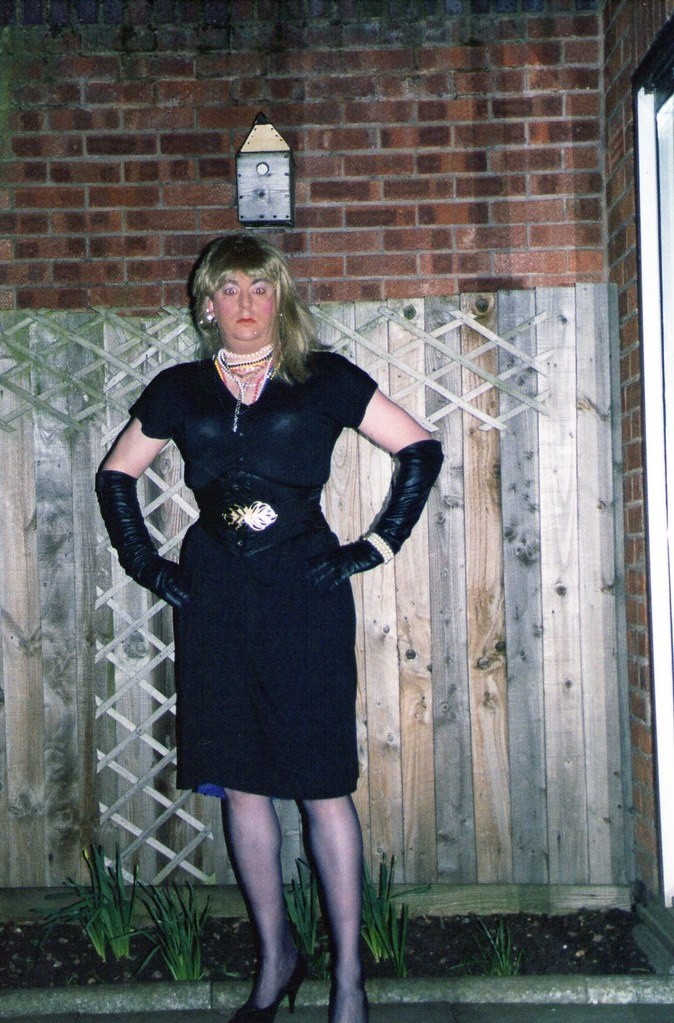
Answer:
[226,949,306,1023]
[329,974,368,1023]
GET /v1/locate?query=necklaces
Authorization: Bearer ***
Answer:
[214,341,283,433]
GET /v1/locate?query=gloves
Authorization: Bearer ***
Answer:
[302,438,445,593]
[94,469,198,608]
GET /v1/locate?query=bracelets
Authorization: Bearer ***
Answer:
[365,533,395,564]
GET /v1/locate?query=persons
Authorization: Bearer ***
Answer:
[94,234,442,1023]
